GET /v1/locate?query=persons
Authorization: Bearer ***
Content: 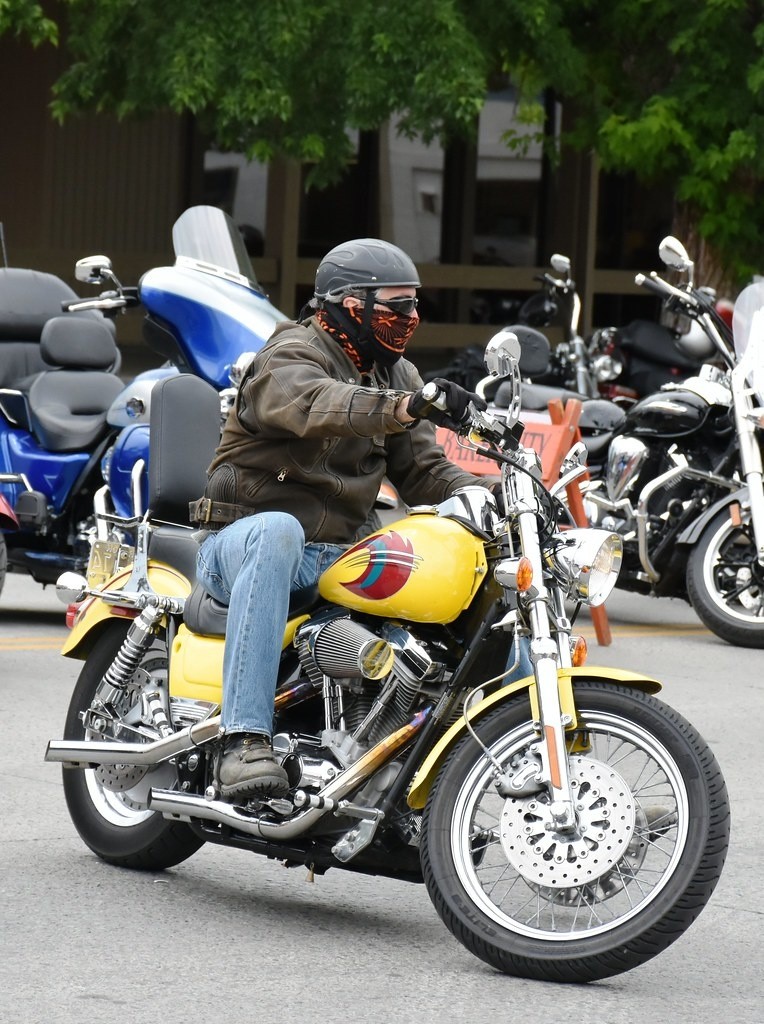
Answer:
[188,238,549,800]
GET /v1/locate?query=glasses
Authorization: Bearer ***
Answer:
[352,295,419,314]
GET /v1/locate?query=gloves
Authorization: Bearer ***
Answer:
[491,484,506,518]
[406,378,488,428]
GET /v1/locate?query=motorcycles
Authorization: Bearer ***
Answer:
[45,335,731,983]
[428,250,736,411]
[474,235,764,649]
[0,206,291,594]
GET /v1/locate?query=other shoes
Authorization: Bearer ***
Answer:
[211,732,289,805]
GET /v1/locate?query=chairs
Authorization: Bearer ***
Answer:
[615,317,700,373]
[492,324,587,412]
[145,369,325,638]
[0,265,123,434]
[28,317,127,454]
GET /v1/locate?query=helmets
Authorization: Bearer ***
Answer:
[315,237,422,297]
